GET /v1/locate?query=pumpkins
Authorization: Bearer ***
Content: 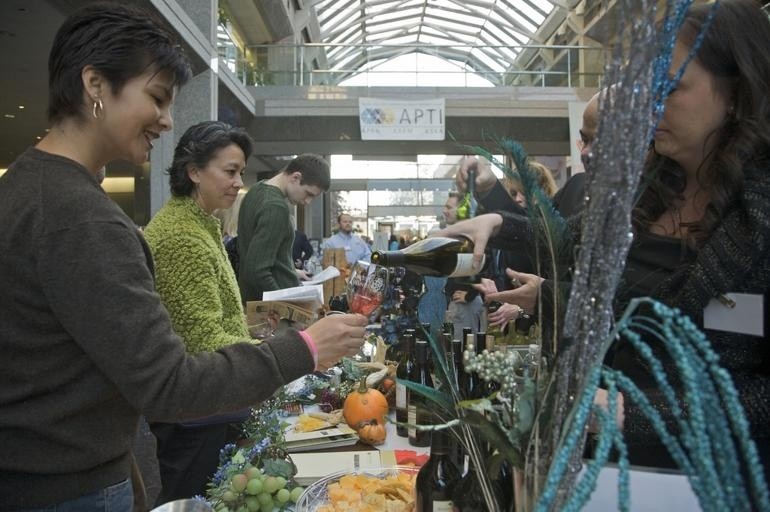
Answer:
[358,419,386,446]
[343,376,389,430]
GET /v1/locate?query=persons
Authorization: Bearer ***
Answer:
[390,1,770,511]
[323,213,373,276]
[289,213,314,270]
[214,208,238,282]
[0,0,369,512]
[142,120,344,502]
[234,152,331,312]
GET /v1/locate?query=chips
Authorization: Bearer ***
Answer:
[316,471,418,512]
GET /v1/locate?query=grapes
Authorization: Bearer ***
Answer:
[214,466,305,512]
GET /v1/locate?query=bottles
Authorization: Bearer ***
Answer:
[371,236,494,278]
[454,169,486,223]
[395,321,547,512]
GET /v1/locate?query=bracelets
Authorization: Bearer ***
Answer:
[297,330,318,375]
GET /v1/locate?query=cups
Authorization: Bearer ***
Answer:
[346,259,390,319]
[303,260,316,278]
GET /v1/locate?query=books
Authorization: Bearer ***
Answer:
[267,411,360,454]
[300,265,341,287]
[283,449,382,487]
[261,282,325,311]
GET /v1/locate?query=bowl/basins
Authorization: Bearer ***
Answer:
[295,466,420,512]
